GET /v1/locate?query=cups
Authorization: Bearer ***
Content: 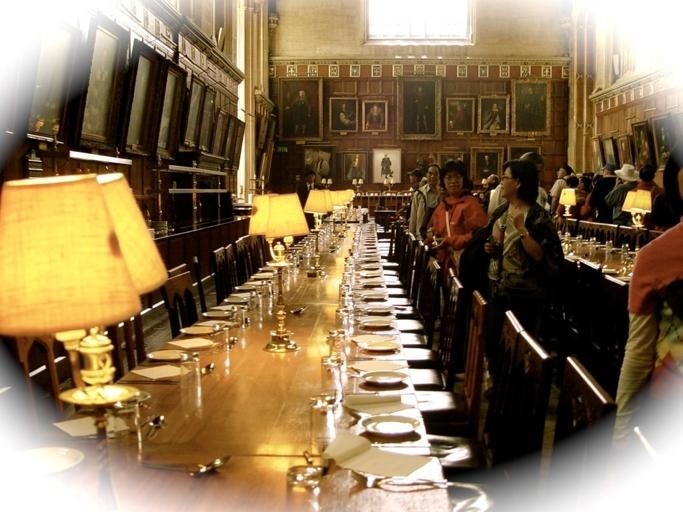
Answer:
[281,269,294,294]
[321,356,343,401]
[336,308,350,336]
[346,224,366,264]
[267,282,278,307]
[339,285,355,312]
[330,205,366,221]
[239,307,250,329]
[285,464,320,512]
[345,264,355,279]
[330,329,347,366]
[180,351,201,417]
[203,311,232,319]
[114,405,142,460]
[210,324,229,354]
[253,293,266,323]
[302,220,334,269]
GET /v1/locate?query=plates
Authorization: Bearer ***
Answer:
[359,305,394,314]
[362,415,420,437]
[224,296,250,304]
[343,273,355,291]
[363,370,407,386]
[360,280,385,287]
[235,283,257,290]
[146,350,182,361]
[360,291,387,300]
[571,238,589,242]
[360,271,383,278]
[251,275,272,280]
[180,326,214,336]
[593,243,609,248]
[582,241,601,245]
[259,267,277,272]
[362,224,379,270]
[359,339,401,353]
[284,232,316,255]
[619,250,638,256]
[601,246,622,251]
[361,319,393,328]
[35,447,82,472]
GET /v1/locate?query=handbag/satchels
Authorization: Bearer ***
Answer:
[450,248,463,276]
[492,272,541,308]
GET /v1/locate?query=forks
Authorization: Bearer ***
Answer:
[351,470,447,489]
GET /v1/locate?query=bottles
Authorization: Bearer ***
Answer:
[563,233,574,255]
[601,240,619,272]
[587,239,604,264]
[573,235,588,259]
[557,231,567,251]
[618,243,635,278]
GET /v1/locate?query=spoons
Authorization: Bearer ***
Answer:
[181,364,215,377]
[222,336,238,350]
[346,410,392,420]
[352,365,367,378]
[142,415,165,442]
[142,454,232,477]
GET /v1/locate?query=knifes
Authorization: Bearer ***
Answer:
[388,478,447,487]
[116,379,179,384]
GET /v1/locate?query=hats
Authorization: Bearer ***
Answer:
[601,164,615,171]
[614,164,639,182]
[408,168,423,175]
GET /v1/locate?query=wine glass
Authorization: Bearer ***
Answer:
[310,394,336,465]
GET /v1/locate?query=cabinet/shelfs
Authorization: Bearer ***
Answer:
[140,164,250,311]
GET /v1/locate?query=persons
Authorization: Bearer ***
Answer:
[451,104,466,130]
[412,86,429,133]
[523,87,546,117]
[401,151,547,331]
[294,170,319,246]
[483,103,504,130]
[610,141,683,456]
[483,158,563,399]
[550,147,681,234]
[336,103,355,129]
[284,90,311,136]
[350,154,363,179]
[379,154,395,178]
[366,103,385,130]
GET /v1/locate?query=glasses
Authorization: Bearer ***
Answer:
[502,175,512,181]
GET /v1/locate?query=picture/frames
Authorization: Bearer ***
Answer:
[340,149,369,183]
[276,76,325,142]
[1,13,246,174]
[468,145,506,183]
[590,111,682,171]
[476,94,510,133]
[507,144,543,159]
[370,144,402,184]
[511,78,553,136]
[361,97,389,135]
[444,96,477,134]
[328,95,359,133]
[437,150,468,167]
[396,75,443,141]
[303,144,336,181]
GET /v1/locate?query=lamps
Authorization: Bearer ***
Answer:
[98,170,168,293]
[0,173,144,407]
